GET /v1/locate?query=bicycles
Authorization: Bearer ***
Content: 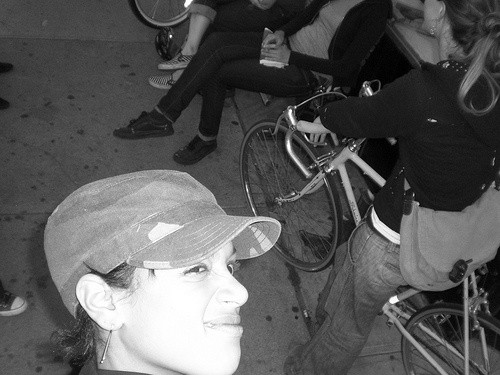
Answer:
[128,0,194,31]
[238,80,499,375]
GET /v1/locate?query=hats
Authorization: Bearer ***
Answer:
[44,170,282,319]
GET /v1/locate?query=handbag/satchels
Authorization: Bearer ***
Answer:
[399,181,499,291]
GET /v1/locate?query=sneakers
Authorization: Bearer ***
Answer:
[114,111,174,139]
[0,290,28,317]
[173,136,217,165]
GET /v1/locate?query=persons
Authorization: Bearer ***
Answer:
[285,0,500,375]
[44,169,282,375]
[113,0,393,164]
[0,281,27,316]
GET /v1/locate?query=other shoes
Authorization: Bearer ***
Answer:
[158,51,194,69]
[148,73,177,90]
[0,62,13,73]
[0,98,9,109]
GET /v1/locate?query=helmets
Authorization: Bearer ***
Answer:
[155,26,181,60]
